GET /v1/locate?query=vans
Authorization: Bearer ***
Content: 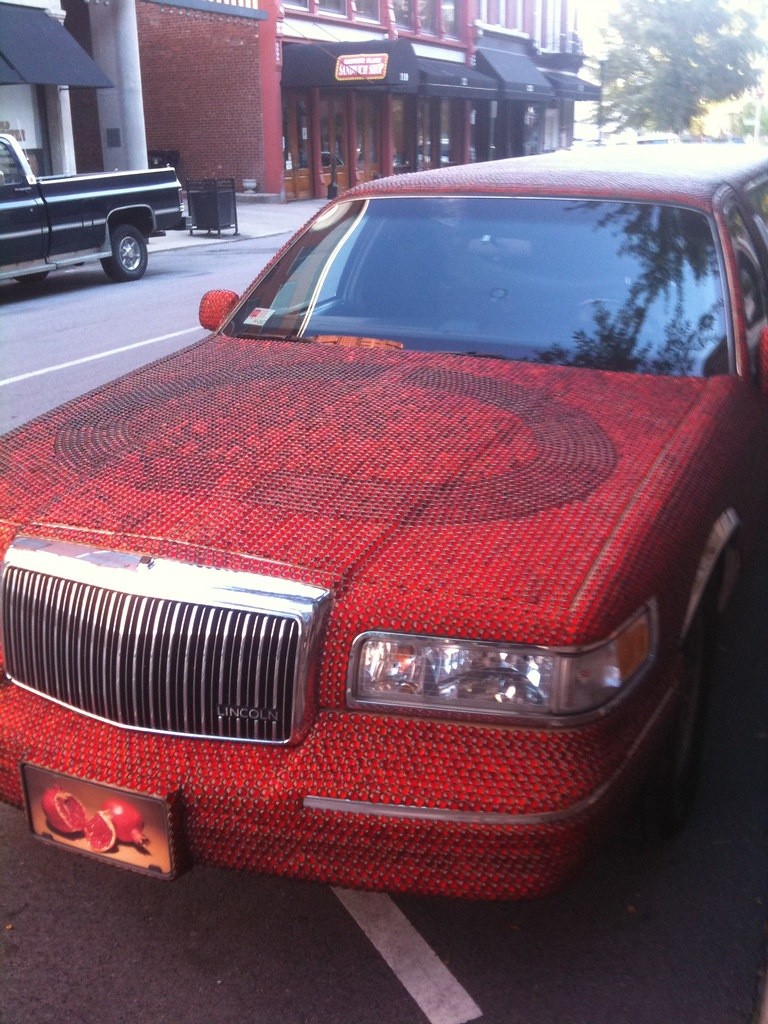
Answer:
[634,133,680,145]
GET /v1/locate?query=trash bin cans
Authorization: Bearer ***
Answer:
[185,176,240,239]
[147,148,180,173]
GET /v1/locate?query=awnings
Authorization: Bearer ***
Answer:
[280,37,601,101]
[0,2,114,91]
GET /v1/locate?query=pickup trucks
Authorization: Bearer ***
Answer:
[0,134,192,285]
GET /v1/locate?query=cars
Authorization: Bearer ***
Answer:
[0,141,768,907]
